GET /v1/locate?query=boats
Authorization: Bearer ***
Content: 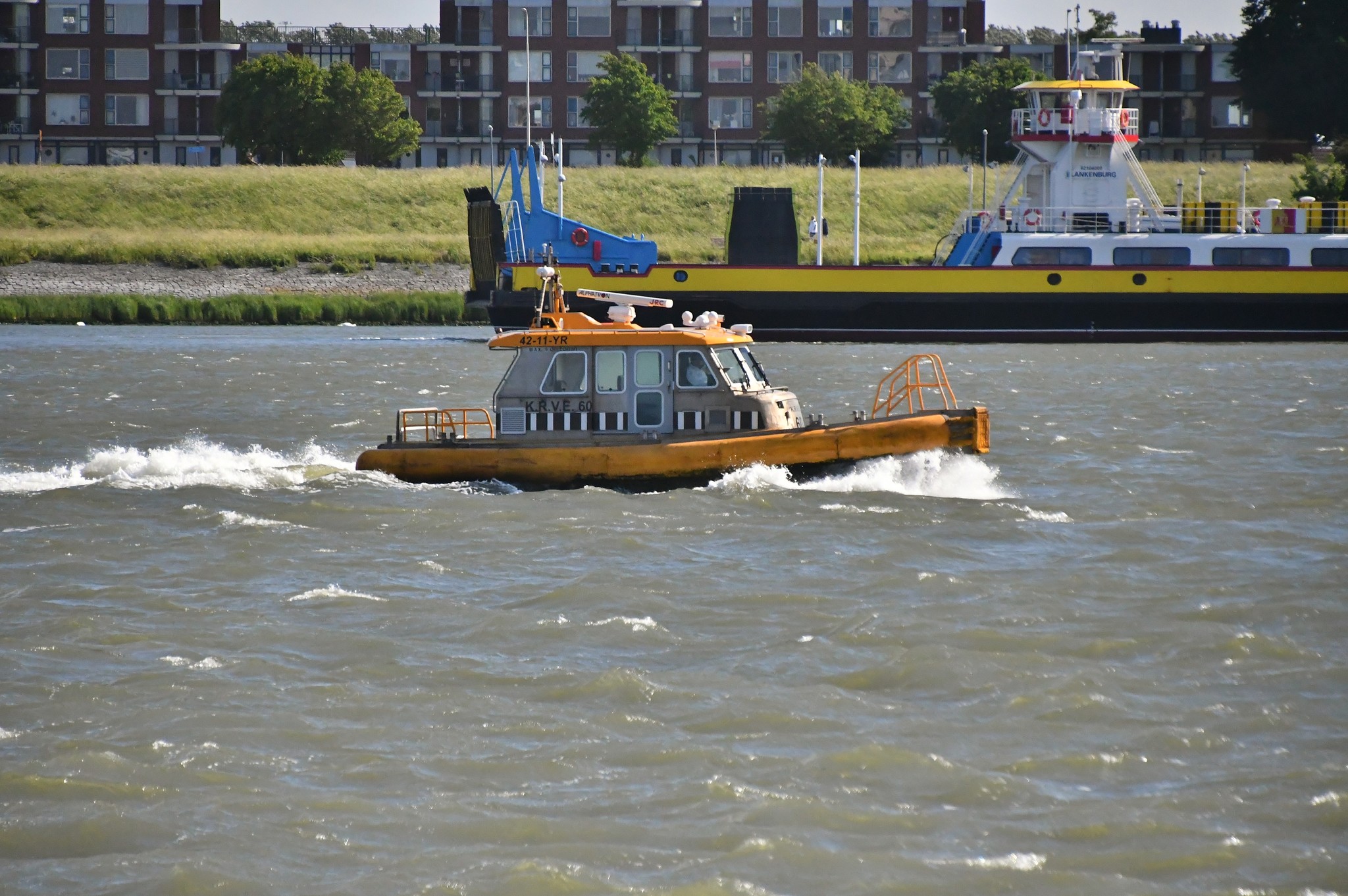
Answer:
[351,249,992,488]
[461,4,1348,343]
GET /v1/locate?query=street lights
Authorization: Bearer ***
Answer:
[711,124,719,166]
[522,6,531,148]
[1065,8,1072,77]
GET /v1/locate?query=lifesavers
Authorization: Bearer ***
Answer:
[1038,108,1050,127]
[1023,209,1042,226]
[1121,110,1129,128]
[977,212,992,228]
[572,227,589,247]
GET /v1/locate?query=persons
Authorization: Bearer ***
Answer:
[686,352,710,386]
